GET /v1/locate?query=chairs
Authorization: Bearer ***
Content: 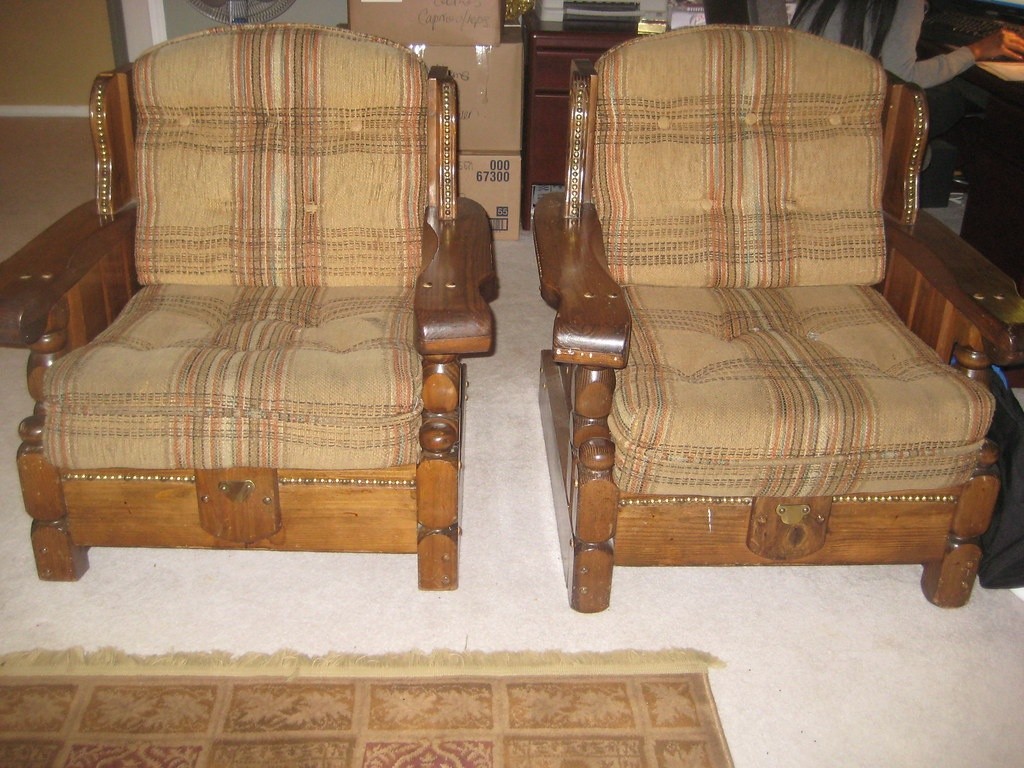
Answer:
[532,24,1024,612]
[0,24,500,590]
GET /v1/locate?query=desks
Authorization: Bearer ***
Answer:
[924,39,1024,297]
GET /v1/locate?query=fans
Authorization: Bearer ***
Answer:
[188,0,296,25]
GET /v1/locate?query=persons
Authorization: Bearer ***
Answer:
[790,0,1024,139]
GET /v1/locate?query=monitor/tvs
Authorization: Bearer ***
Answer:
[950,0,1024,20]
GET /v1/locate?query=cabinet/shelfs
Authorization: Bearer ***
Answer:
[524,12,647,239]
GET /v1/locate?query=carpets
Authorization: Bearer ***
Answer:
[0,643,737,768]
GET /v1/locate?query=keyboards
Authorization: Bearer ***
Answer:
[919,11,1024,45]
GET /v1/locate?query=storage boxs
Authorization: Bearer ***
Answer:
[457,152,522,241]
[407,20,524,152]
[348,0,503,45]
[638,16,669,35]
